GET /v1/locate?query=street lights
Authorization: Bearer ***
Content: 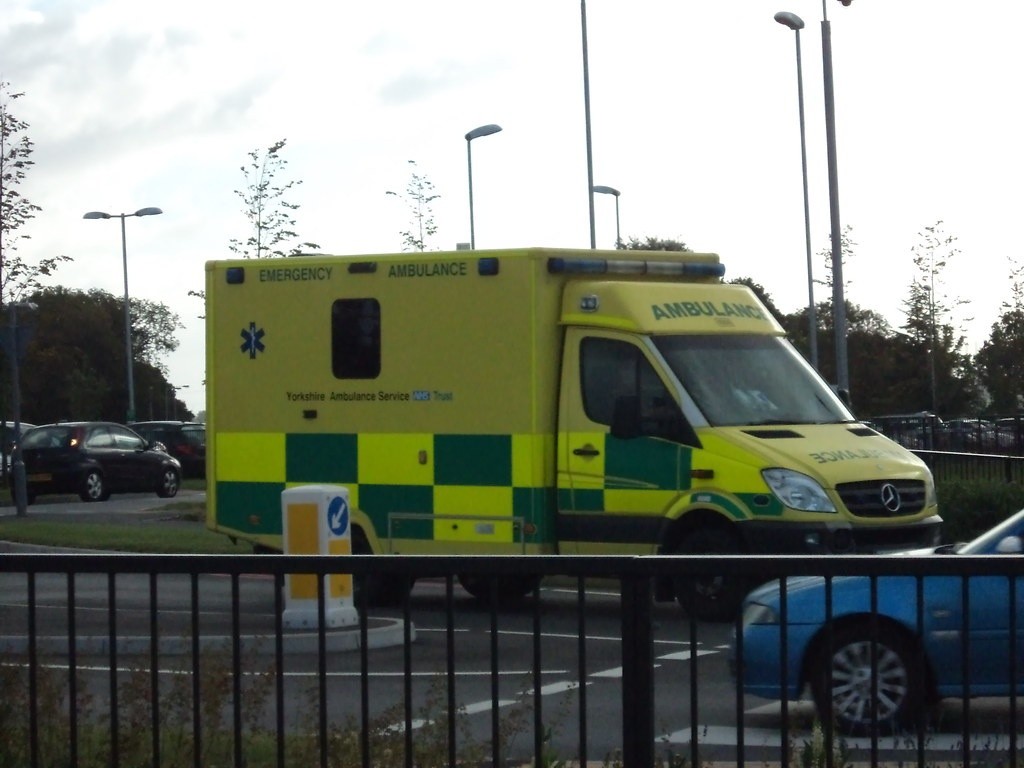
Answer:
[466,125,502,250]
[83,208,162,425]
[821,0,853,401]
[592,186,620,252]
[775,13,819,371]
[924,285,936,411]
[166,385,189,421]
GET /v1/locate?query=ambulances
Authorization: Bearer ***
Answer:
[207,251,942,622]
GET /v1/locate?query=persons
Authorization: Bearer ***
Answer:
[613,349,708,449]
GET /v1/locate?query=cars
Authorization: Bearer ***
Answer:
[0,421,206,502]
[726,509,1024,737]
[859,409,1024,451]
